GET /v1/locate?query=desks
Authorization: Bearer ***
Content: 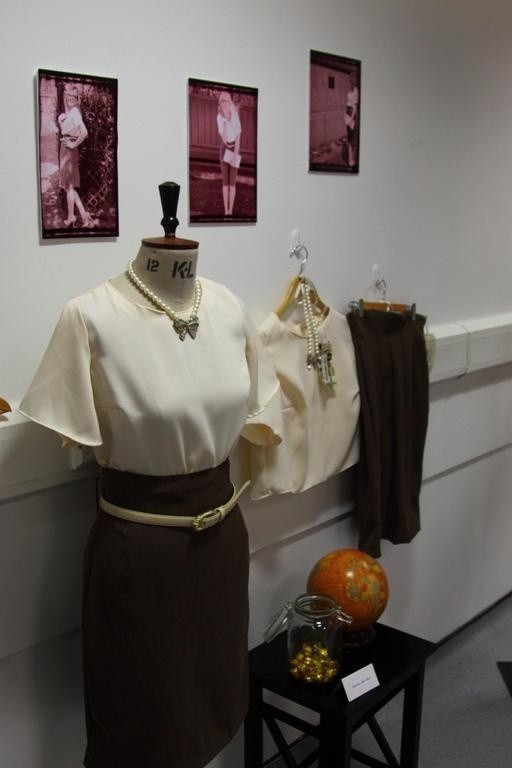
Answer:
[242,615,438,767]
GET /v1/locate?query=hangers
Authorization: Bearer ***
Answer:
[250,244,350,346]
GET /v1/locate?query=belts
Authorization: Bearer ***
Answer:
[96,478,252,533]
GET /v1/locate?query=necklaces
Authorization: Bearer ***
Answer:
[300,284,322,371]
[127,257,202,341]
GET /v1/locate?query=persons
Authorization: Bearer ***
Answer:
[58,89,92,227]
[18,237,285,768]
[216,91,242,216]
[344,86,359,167]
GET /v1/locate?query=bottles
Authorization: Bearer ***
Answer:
[265,594,351,687]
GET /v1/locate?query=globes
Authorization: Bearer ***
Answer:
[306,548,390,648]
